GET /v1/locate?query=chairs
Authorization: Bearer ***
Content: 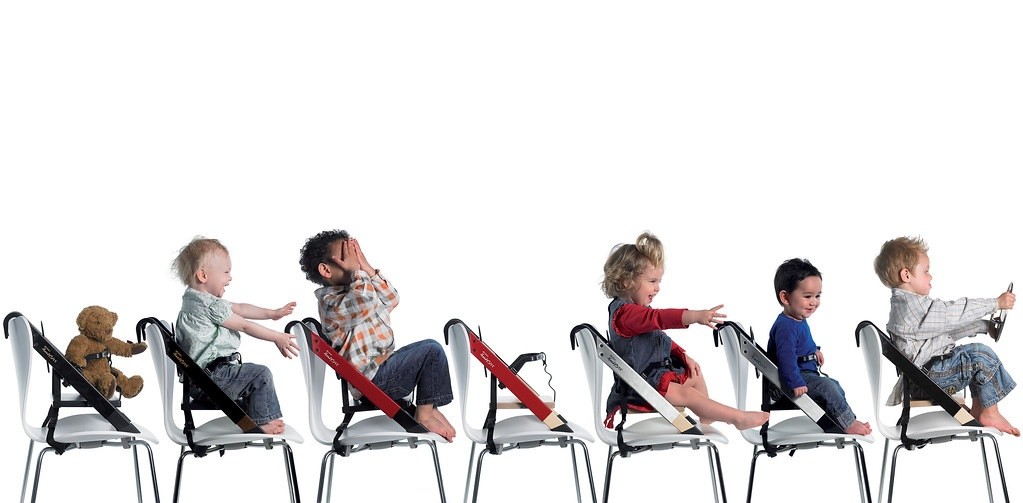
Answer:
[8,316,1010,503]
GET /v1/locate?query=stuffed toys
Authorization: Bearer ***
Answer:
[63,305,149,399]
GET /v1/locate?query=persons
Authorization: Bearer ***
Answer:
[171,236,301,434]
[598,232,770,430]
[873,236,1021,437]
[763,258,873,436]
[299,229,456,443]
